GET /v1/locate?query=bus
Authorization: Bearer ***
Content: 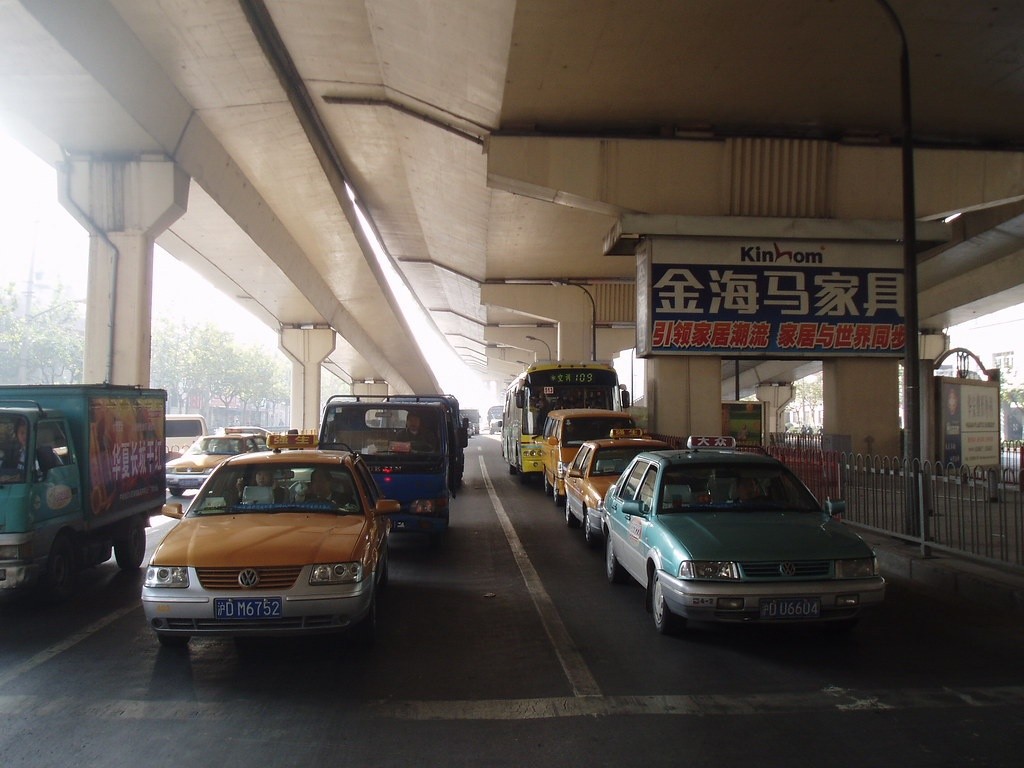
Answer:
[459,409,481,435]
[487,405,504,435]
[501,358,630,485]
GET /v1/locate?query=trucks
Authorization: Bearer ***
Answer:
[319,395,469,535]
[0,383,167,613]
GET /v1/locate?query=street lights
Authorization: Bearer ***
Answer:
[550,279,596,362]
[526,335,551,360]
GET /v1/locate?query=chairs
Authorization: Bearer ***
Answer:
[595,459,617,470]
[37,428,56,449]
[339,429,379,449]
[663,483,691,508]
[708,476,734,504]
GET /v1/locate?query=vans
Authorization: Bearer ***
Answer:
[541,409,636,508]
[166,415,210,456]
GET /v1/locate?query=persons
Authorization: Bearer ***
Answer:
[8,420,57,482]
[236,470,289,504]
[306,469,347,507]
[393,412,433,452]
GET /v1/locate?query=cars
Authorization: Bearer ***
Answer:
[563,428,675,547]
[165,433,273,496]
[140,434,401,647]
[601,436,887,636]
